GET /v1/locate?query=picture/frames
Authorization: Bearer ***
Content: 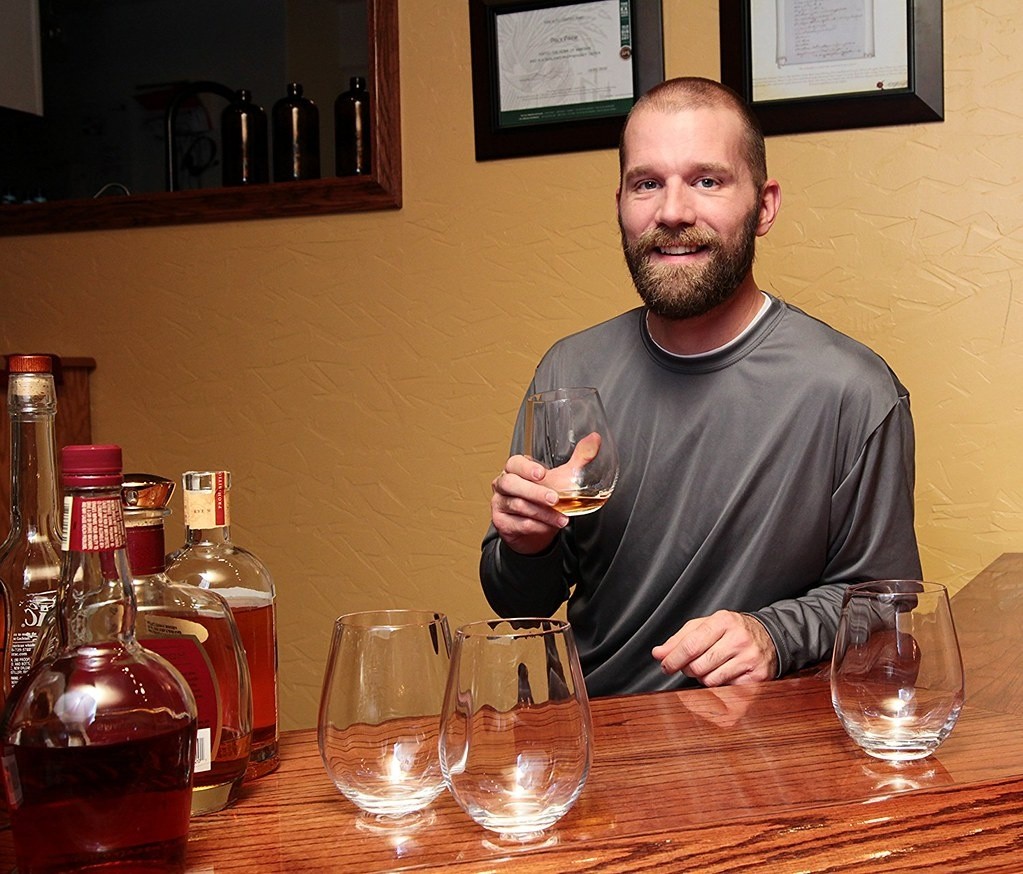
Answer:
[469,0,665,161]
[718,0,945,137]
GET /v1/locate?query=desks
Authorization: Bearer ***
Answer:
[0,552,1023,874]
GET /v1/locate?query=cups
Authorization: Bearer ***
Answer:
[438,617,590,841]
[830,579,964,760]
[524,384,620,517]
[316,609,471,816]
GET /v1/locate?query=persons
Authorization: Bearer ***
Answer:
[479,76,925,697]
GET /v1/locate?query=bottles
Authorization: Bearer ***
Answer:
[112,471,253,818]
[271,83,320,182]
[0,352,67,718]
[0,444,199,874]
[161,469,281,785]
[220,87,270,188]
[332,77,372,177]
